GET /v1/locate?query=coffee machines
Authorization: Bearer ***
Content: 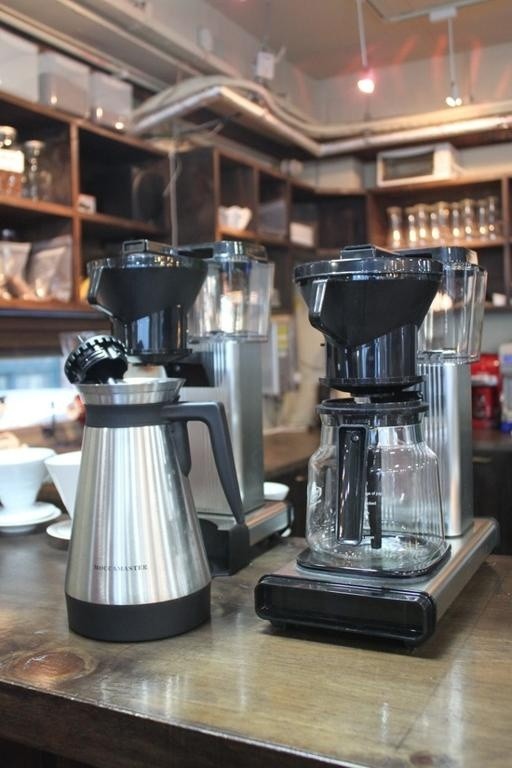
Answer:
[254,244,502,648]
[86,239,297,578]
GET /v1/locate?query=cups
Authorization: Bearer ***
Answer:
[44,449,83,523]
[0,446,55,512]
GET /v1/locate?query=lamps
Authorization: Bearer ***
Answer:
[445,17,463,108]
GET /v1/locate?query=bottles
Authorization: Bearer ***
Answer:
[0,124,54,203]
[385,196,504,249]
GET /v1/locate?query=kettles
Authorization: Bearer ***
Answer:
[64,334,246,644]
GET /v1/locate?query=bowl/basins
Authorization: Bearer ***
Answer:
[218,207,254,232]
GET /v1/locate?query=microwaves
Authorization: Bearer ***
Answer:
[377,141,463,188]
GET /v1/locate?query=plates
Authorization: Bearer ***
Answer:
[45,521,74,541]
[0,501,62,534]
